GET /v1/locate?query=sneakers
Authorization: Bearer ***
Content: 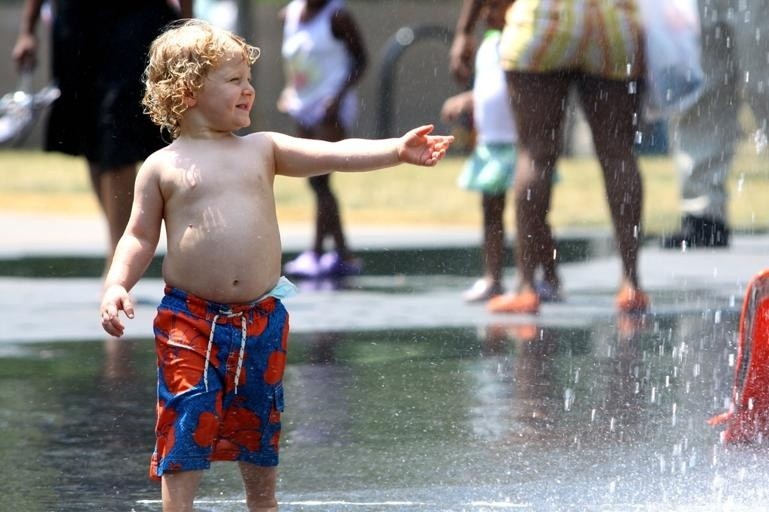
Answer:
[462,279,503,302]
[617,289,650,312]
[283,250,322,280]
[660,215,728,247]
[535,278,560,300]
[486,291,540,315]
[319,247,365,274]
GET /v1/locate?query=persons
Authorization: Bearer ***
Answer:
[464,0,657,313]
[55,343,171,512]
[97,17,459,511]
[270,2,371,283]
[11,0,181,271]
[472,320,655,498]
[449,0,512,78]
[189,0,260,54]
[281,327,357,462]
[440,27,566,305]
[663,1,769,247]
